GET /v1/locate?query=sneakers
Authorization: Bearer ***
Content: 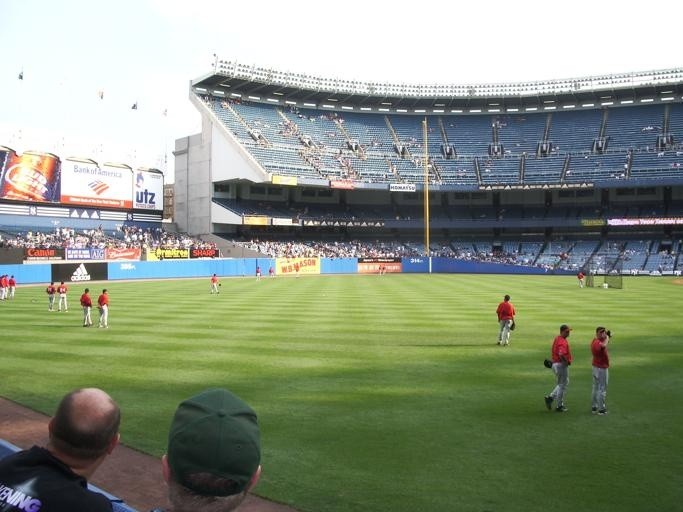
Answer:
[556,405,569,412]
[592,406,598,415]
[598,409,608,416]
[544,396,554,411]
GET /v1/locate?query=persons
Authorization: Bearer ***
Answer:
[150,389,262,512]
[46,282,56,312]
[0,387,121,511]
[97,289,109,328]
[80,288,93,327]
[544,325,572,412]
[590,326,610,415]
[496,295,515,346]
[57,281,68,312]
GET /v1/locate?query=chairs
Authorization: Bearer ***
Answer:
[212,198,683,227]
[197,89,683,181]
[447,236,682,273]
[211,233,457,258]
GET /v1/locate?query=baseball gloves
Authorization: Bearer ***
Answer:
[606,331,612,338]
[511,323,516,330]
[544,359,552,368]
[218,284,221,287]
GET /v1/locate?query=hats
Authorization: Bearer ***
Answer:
[503,295,510,300]
[166,387,262,485]
[559,325,573,331]
[597,327,606,332]
